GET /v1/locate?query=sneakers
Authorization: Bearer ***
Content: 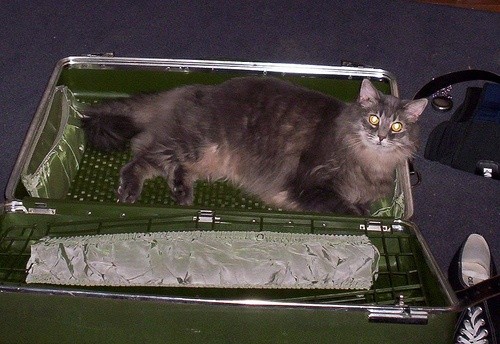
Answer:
[450,232,498,343]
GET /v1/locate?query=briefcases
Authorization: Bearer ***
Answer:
[1,54,461,342]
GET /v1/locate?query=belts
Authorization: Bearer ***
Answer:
[404,68,500,188]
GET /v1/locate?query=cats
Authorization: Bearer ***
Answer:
[73,75,429,217]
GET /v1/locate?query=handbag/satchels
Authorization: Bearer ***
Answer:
[423,81,500,180]
